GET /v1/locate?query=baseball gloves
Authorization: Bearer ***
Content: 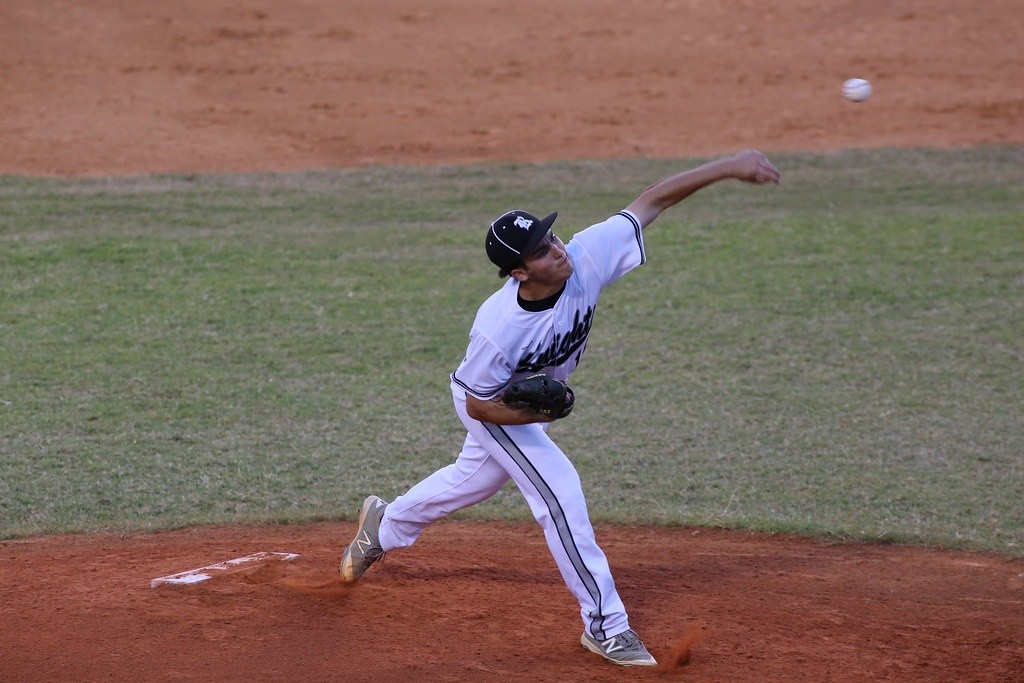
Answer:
[503,373,576,420]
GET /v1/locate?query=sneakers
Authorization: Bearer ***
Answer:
[339,495,390,585]
[581,629,658,666]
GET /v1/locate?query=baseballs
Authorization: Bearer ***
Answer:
[842,78,873,103]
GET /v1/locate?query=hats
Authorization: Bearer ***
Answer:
[484,210,560,278]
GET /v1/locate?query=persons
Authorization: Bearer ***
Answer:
[342,148,779,666]
